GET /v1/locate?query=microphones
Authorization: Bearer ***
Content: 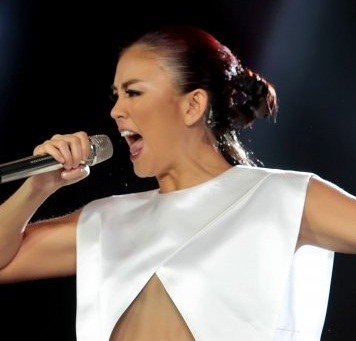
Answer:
[0,135,114,184]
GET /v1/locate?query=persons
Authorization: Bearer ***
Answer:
[0,27,356,341]
